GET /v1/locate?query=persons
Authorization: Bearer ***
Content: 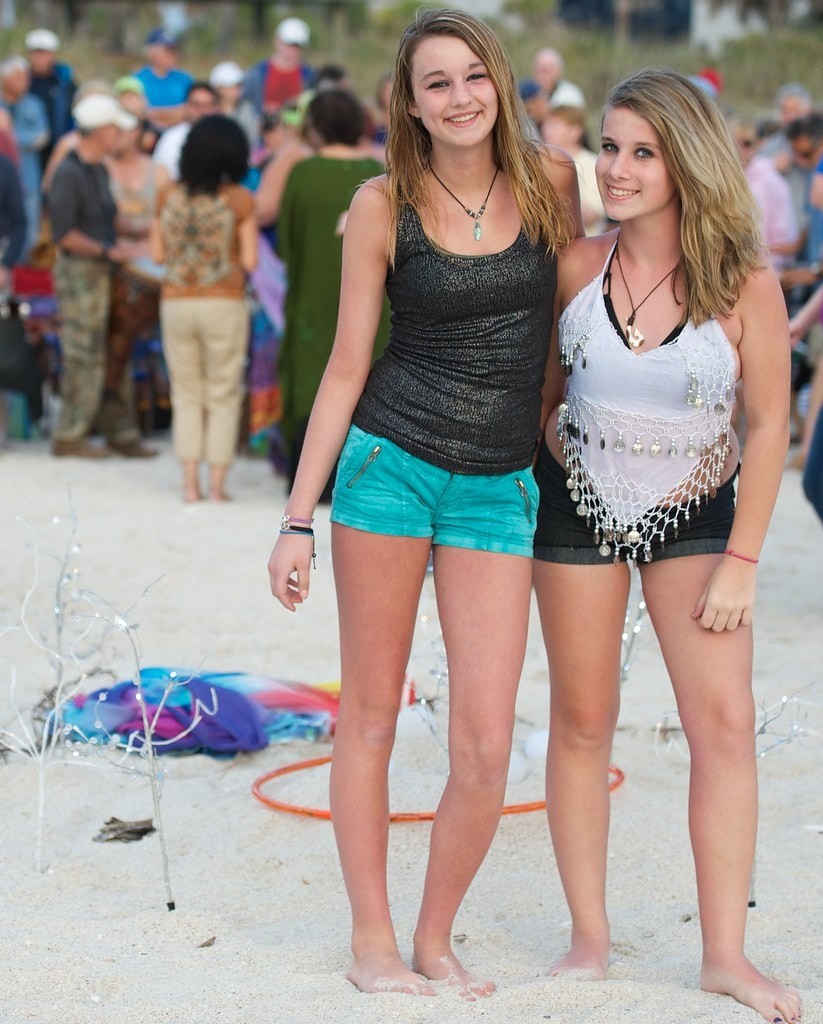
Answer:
[535,64,810,1024]
[2,0,820,521]
[262,9,592,1003]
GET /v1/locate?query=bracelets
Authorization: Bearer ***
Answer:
[280,512,314,524]
[723,549,758,564]
[278,529,318,569]
[281,522,313,534]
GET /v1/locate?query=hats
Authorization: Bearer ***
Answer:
[275,17,312,47]
[72,93,139,132]
[208,59,247,90]
[144,27,181,47]
[549,81,588,112]
[25,28,61,53]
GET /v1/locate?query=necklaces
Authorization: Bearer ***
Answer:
[425,157,501,241]
[613,229,683,351]
